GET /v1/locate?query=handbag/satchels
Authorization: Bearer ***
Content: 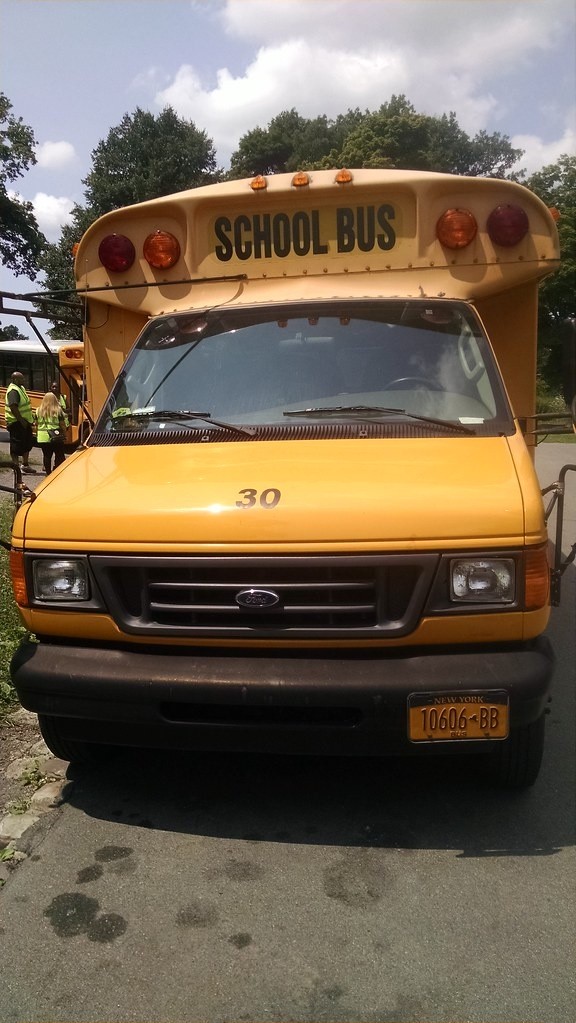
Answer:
[47,428,66,442]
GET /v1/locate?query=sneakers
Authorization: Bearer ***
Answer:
[20,465,36,473]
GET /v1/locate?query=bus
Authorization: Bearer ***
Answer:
[0,338,91,448]
[0,164,576,791]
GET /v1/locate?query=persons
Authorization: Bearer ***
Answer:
[35,382,71,477]
[5,372,36,473]
